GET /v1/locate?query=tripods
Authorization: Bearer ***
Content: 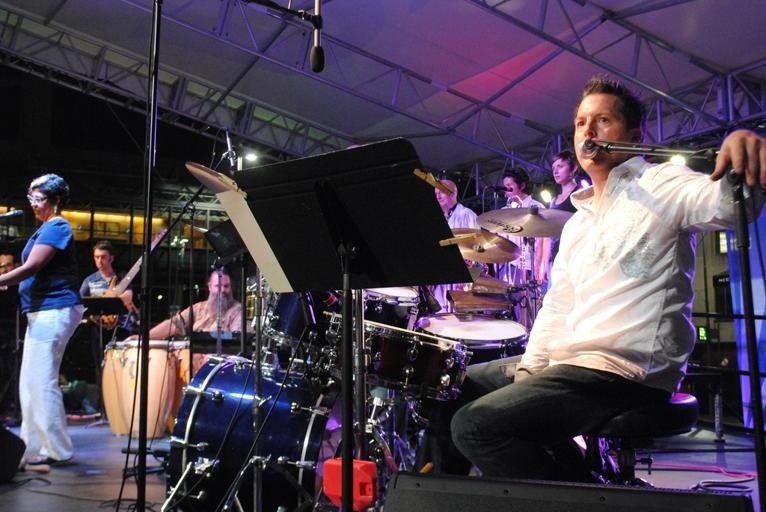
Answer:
[366,385,416,474]
[85,314,111,429]
[313,289,398,512]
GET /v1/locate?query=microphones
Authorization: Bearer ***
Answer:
[488,185,513,192]
[579,139,598,159]
[224,130,236,170]
[310,0,324,73]
[0,210,23,219]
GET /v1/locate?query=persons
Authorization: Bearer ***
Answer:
[426,171,477,322]
[541,143,593,283]
[2,253,24,289]
[2,173,84,470]
[478,158,544,330]
[124,267,246,429]
[442,79,765,481]
[79,246,140,341]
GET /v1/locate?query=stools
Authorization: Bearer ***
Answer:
[577,392,700,491]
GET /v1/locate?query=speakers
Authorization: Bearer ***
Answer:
[382,470,756,512]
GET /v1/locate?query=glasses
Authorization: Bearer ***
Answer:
[27,195,48,203]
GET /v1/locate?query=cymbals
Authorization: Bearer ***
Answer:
[476,208,574,237]
[450,228,522,263]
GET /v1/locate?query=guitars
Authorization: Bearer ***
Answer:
[89,227,168,331]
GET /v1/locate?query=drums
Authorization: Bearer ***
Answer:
[101,341,185,440]
[166,353,397,511]
[250,274,351,354]
[354,288,419,339]
[412,312,529,433]
[310,311,474,402]
[168,345,212,434]
